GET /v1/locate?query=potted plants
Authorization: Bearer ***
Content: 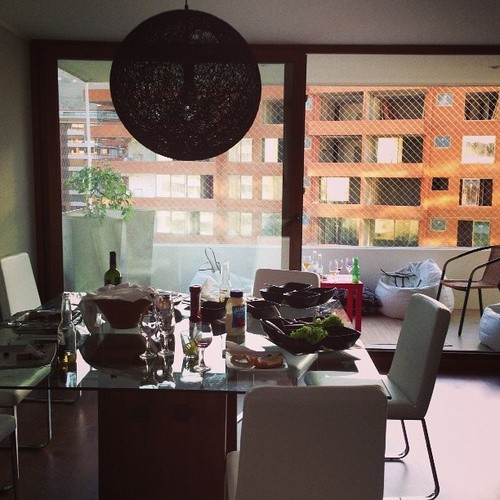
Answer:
[64,165,135,289]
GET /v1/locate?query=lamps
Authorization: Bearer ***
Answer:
[109,0,262,161]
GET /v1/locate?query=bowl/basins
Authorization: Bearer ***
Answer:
[321,326,361,351]
[280,282,312,292]
[92,299,152,330]
[307,287,336,304]
[260,317,302,335]
[260,286,285,303]
[283,290,321,309]
[298,316,329,324]
[200,301,226,319]
[276,329,323,355]
[246,300,281,319]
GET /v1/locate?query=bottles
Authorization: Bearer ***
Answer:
[57,292,77,364]
[219,262,234,303]
[312,250,323,283]
[104,251,123,286]
[225,290,246,348]
[352,256,360,283]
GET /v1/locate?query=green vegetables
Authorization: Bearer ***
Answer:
[290,315,343,345]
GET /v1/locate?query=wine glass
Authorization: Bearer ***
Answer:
[346,256,353,281]
[140,313,159,356]
[302,256,311,271]
[156,311,175,355]
[192,322,213,371]
[328,259,344,281]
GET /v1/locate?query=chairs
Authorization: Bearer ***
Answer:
[0,364,52,492]
[225,384,387,500]
[436,244,500,337]
[253,269,320,297]
[380,292,451,500]
[0,252,41,319]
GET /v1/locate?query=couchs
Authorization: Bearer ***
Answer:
[374,259,454,319]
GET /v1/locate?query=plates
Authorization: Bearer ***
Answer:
[225,352,289,371]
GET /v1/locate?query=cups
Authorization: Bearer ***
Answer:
[181,330,199,358]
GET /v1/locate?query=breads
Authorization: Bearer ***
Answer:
[257,355,283,368]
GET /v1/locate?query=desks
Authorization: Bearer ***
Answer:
[319,274,364,332]
[0,296,392,500]
